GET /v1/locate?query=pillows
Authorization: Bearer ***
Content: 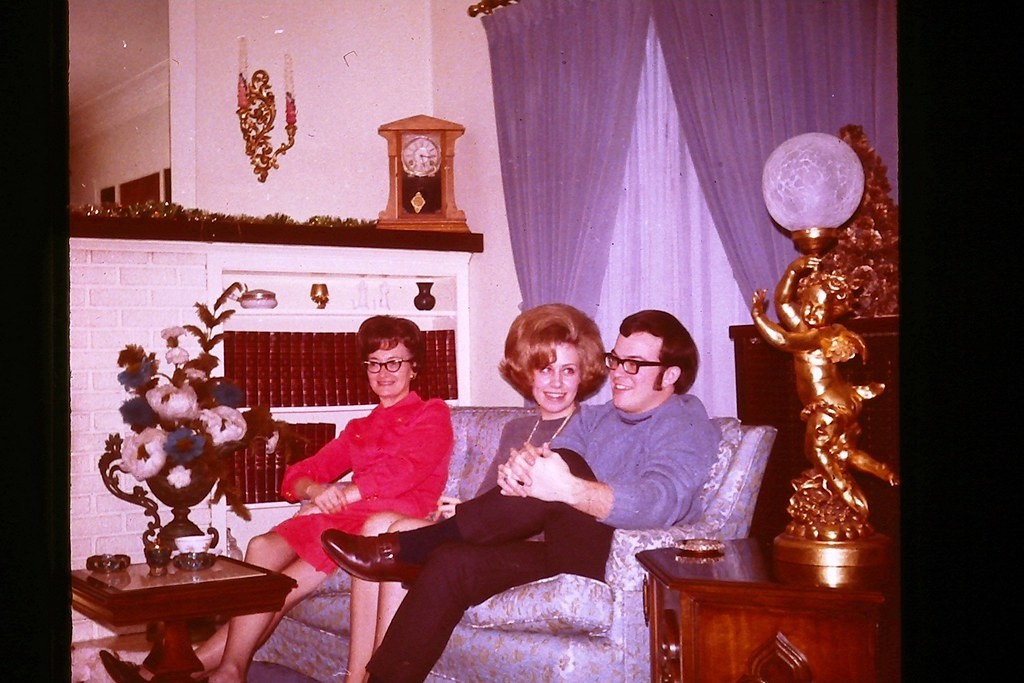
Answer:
[681,412,743,525]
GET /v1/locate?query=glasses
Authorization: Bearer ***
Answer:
[364,359,410,373]
[604,353,671,374]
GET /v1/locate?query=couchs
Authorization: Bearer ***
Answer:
[248,405,781,683]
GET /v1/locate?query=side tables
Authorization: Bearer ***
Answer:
[630,534,880,683]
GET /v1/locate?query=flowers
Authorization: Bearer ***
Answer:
[96,281,279,483]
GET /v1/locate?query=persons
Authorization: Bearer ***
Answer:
[99,315,455,683]
[322,310,720,683]
[342,303,610,683]
[751,254,902,514]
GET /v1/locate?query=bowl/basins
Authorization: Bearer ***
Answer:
[175,535,212,554]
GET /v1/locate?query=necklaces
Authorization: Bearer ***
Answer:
[526,407,576,445]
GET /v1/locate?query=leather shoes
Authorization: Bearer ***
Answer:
[322,528,423,589]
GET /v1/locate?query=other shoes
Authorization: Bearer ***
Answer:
[99,650,154,683]
[152,670,208,683]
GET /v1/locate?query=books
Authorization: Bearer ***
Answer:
[224,330,457,409]
[226,421,336,507]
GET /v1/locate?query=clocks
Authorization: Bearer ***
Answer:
[400,136,443,176]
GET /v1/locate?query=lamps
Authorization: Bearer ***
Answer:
[232,37,299,182]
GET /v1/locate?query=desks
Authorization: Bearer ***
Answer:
[71,555,298,683]
